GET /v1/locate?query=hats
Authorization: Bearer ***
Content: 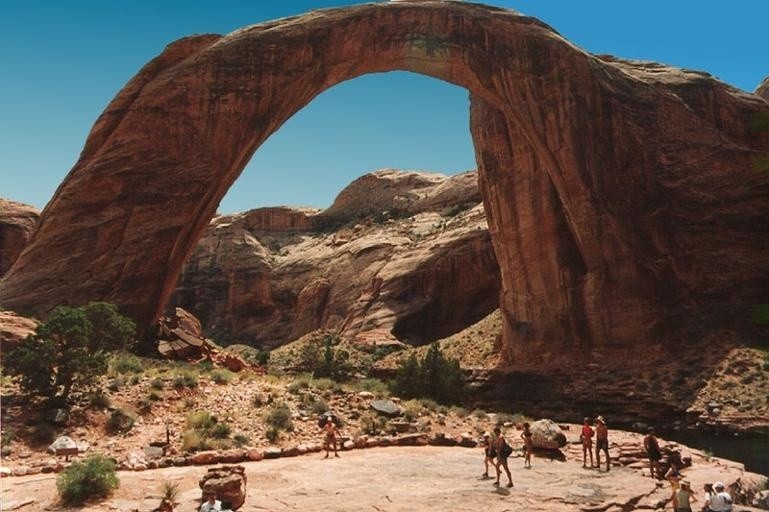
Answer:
[594,415,606,425]
[677,477,690,486]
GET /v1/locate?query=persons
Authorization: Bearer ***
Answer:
[593,416,611,471]
[664,460,687,501]
[319,416,342,458]
[672,480,697,511]
[200,491,222,511]
[699,483,721,512]
[519,422,534,466]
[713,482,733,511]
[643,426,661,476]
[580,417,595,466]
[491,428,514,487]
[218,501,234,511]
[480,430,503,477]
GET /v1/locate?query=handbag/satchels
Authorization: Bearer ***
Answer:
[485,444,497,457]
[498,436,514,460]
